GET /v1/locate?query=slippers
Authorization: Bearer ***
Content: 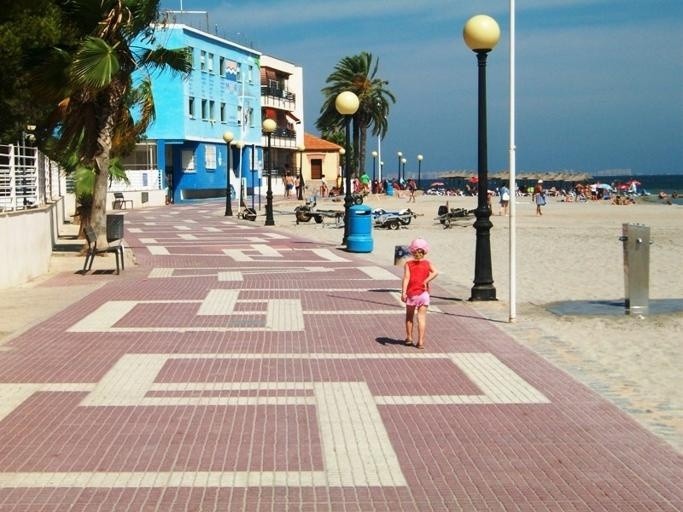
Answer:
[405,339,425,350]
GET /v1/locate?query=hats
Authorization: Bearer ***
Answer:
[410,239,430,253]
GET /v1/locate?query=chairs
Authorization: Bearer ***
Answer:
[83,225,124,275]
[113,193,133,210]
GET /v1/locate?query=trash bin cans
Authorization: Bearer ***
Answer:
[106,215,123,241]
[386,183,393,195]
[346,205,373,253]
[142,192,147,203]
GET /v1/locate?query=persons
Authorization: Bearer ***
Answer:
[400,237,440,350]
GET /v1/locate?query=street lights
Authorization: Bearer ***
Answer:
[223,130,234,216]
[298,144,306,199]
[262,119,277,224]
[335,90,423,245]
[236,140,245,207]
[461,14,500,301]
